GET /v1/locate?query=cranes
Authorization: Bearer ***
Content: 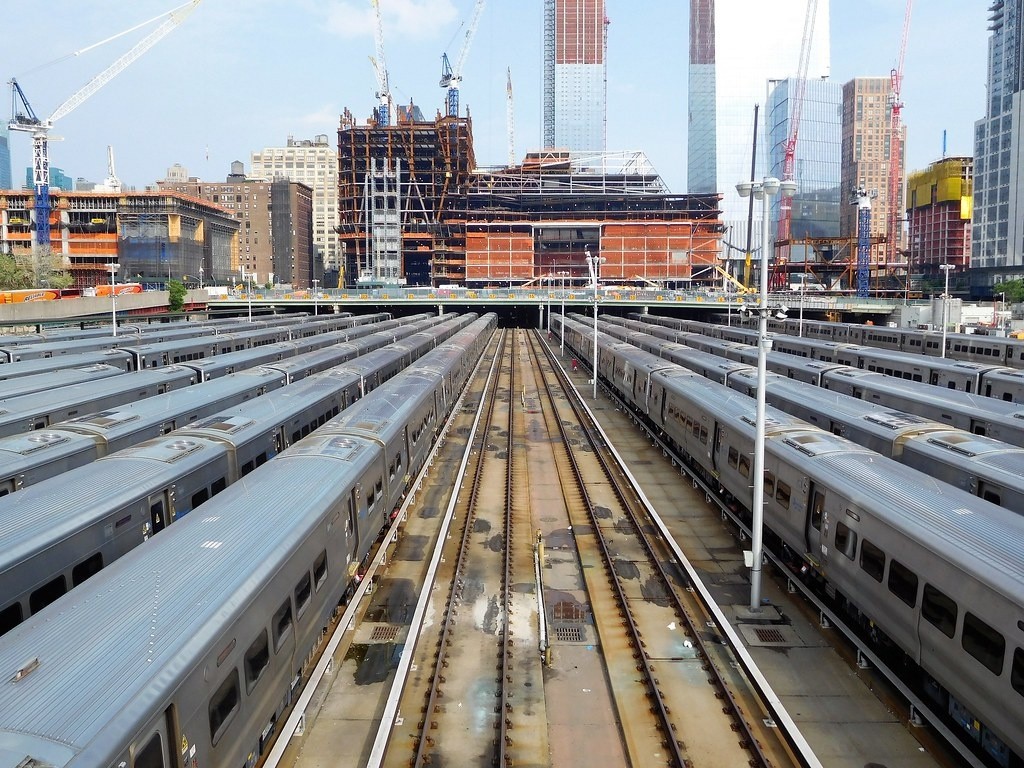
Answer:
[773,0,822,290]
[5,0,207,297]
[369,0,398,143]
[506,65,517,169]
[439,0,487,164]
[882,0,911,276]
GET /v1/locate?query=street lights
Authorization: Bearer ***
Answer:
[586,256,607,399]
[243,273,255,322]
[112,262,122,336]
[312,279,320,315]
[557,271,569,358]
[998,291,1005,331]
[733,178,799,610]
[939,264,955,357]
[797,274,808,336]
[199,257,205,290]
